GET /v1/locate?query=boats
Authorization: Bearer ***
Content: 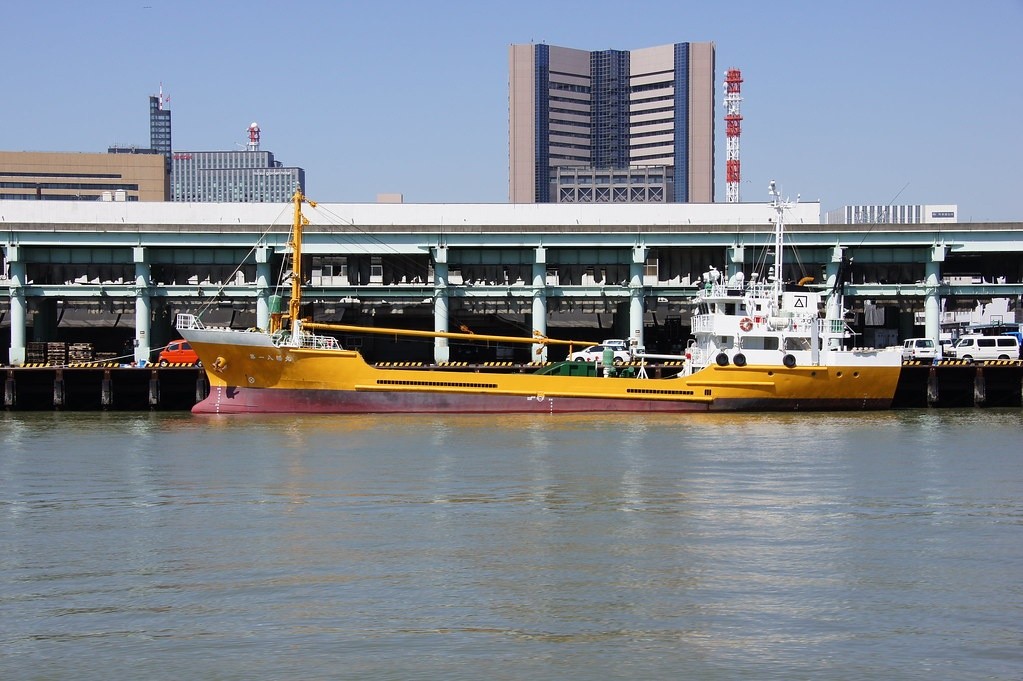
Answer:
[174,179,897,414]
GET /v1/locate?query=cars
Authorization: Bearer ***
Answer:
[566,343,630,365]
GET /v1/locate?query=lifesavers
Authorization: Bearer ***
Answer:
[740,318,752,331]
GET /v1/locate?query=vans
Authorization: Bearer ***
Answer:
[901,338,935,364]
[939,339,952,355]
[159,340,202,367]
[947,335,1019,364]
